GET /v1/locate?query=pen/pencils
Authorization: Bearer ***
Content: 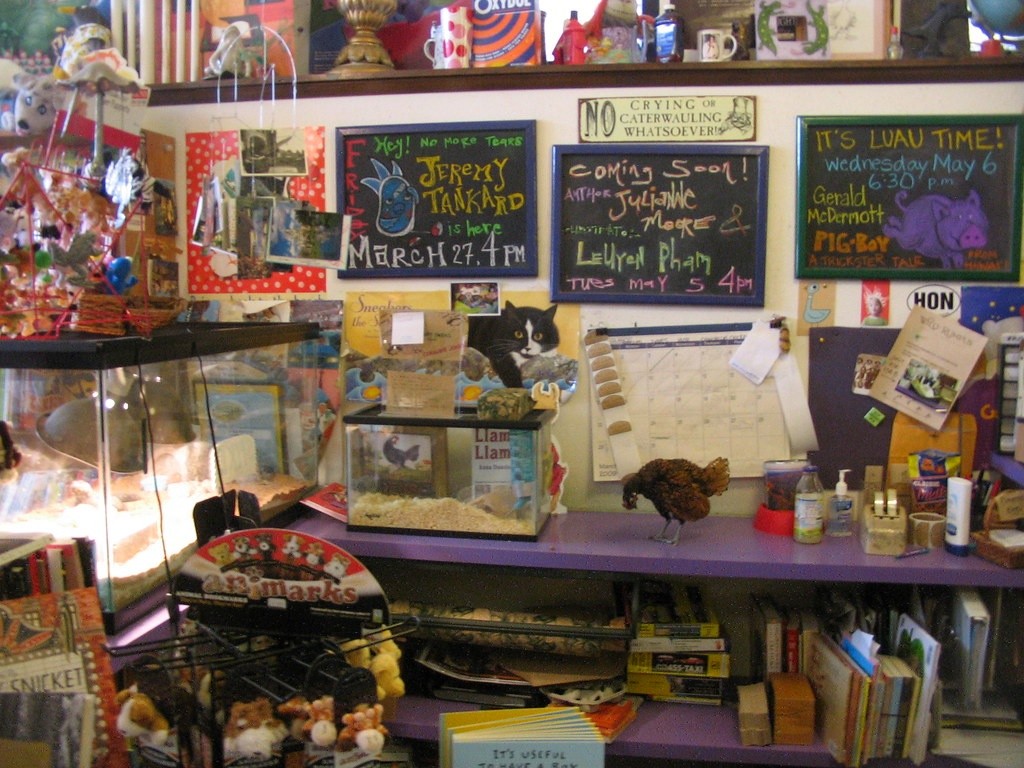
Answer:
[896,548,928,559]
[972,479,1001,512]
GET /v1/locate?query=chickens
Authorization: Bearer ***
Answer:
[620,456,730,546]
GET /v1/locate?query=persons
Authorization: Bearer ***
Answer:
[862,297,887,326]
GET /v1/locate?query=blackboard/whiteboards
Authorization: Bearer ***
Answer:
[334,120,538,279]
[796,114,1024,280]
[547,143,769,307]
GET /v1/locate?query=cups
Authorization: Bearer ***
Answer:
[424,25,446,69]
[696,29,738,62]
[440,6,472,69]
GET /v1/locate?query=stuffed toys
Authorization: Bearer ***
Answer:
[113,610,406,767]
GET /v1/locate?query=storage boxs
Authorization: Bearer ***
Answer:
[342,405,557,543]
[470,0,542,67]
[767,672,817,747]
[0,321,322,635]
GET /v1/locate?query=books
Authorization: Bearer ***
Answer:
[417,585,991,768]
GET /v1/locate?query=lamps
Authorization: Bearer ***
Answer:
[33,371,145,475]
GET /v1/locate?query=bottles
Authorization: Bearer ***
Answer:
[654,5,685,63]
[563,10,586,64]
[793,466,824,544]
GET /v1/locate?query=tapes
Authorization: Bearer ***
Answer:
[873,489,885,515]
[887,488,897,515]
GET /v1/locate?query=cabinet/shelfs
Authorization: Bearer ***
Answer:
[278,453,1024,768]
